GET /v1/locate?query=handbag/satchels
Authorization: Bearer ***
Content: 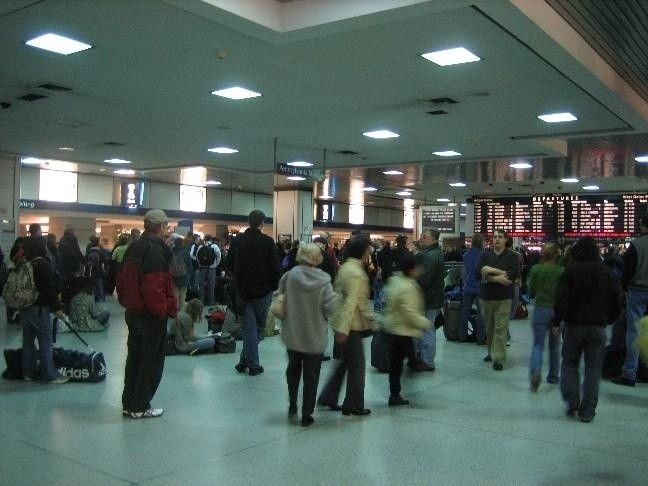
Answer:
[269,294,288,319]
[51,315,107,383]
[1,347,42,378]
[356,304,382,339]
[515,301,530,321]
[206,306,227,335]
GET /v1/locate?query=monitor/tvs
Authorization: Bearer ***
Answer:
[419,206,459,238]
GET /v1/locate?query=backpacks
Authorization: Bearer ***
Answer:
[163,330,178,356]
[197,243,217,267]
[264,308,280,338]
[596,271,621,326]
[2,256,49,311]
[223,277,247,316]
[170,251,190,278]
[211,332,237,354]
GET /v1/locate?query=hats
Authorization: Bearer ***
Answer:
[319,231,335,240]
[143,209,172,225]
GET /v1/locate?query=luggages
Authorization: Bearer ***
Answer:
[444,301,464,341]
[68,292,97,331]
[370,330,389,371]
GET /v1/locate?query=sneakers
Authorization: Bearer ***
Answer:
[407,360,435,372]
[342,408,372,416]
[317,397,342,411]
[288,404,298,417]
[122,407,166,418]
[611,376,638,388]
[483,354,504,371]
[44,375,70,384]
[547,372,559,386]
[301,415,314,427]
[248,367,265,376]
[387,395,411,406]
[565,400,594,421]
[529,374,543,390]
[235,363,246,373]
